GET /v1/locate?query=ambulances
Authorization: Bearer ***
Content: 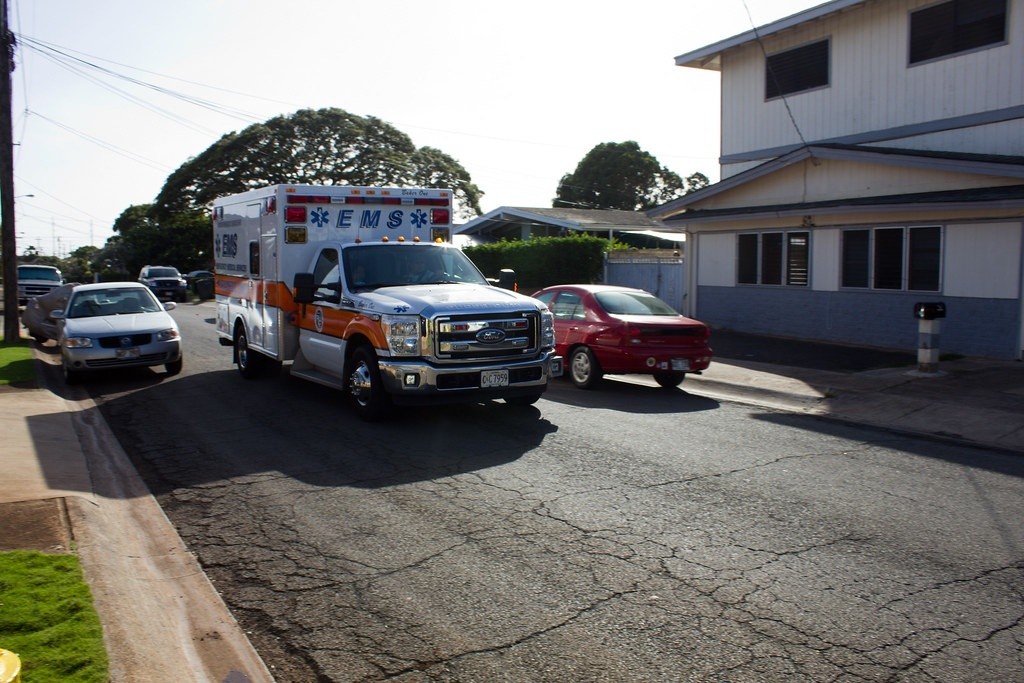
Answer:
[211,184,555,424]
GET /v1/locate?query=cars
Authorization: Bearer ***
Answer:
[17,265,64,306]
[186,271,215,289]
[529,284,713,389]
[48,281,183,389]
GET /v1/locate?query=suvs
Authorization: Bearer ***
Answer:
[137,265,188,302]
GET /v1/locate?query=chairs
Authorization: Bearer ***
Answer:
[79,298,138,316]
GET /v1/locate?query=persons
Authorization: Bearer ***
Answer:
[403,252,453,284]
